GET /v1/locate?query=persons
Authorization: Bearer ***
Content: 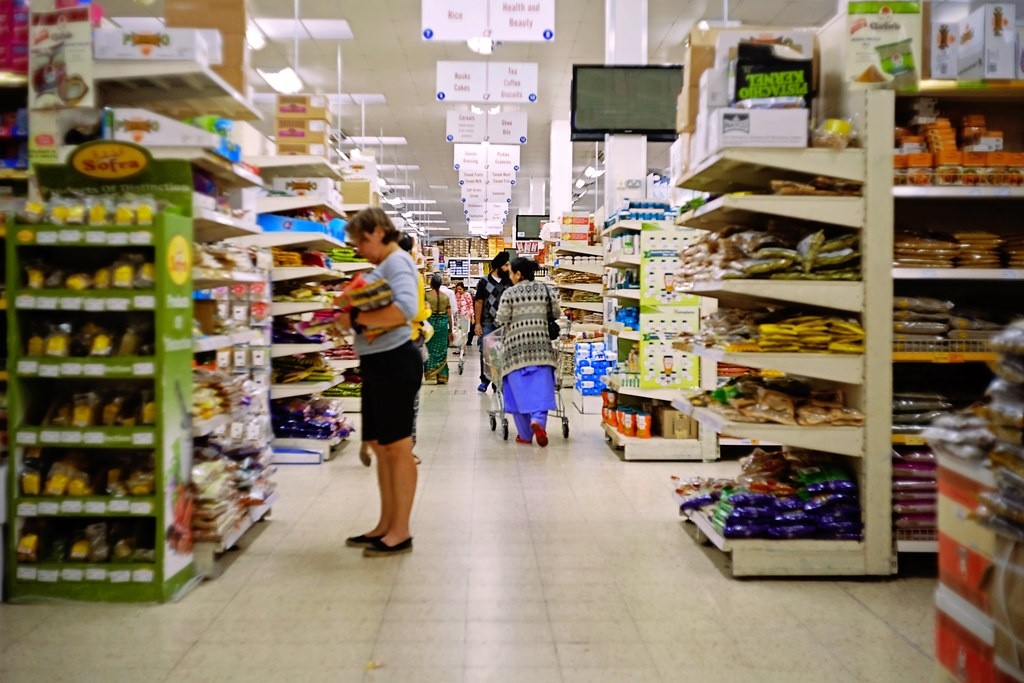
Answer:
[424,274,455,383]
[438,276,457,370]
[333,204,424,557]
[452,282,475,356]
[494,257,561,447]
[474,252,513,393]
[360,231,432,467]
[466,287,475,346]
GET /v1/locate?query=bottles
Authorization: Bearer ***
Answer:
[611,231,641,255]
[602,388,651,440]
[602,269,639,290]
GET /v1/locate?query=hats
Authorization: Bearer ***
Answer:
[491,251,510,269]
[441,277,450,284]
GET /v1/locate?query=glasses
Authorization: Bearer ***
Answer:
[352,229,375,248]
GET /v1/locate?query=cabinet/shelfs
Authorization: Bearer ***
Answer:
[259,154,350,460]
[554,219,718,460]
[0,0,276,604]
[446,254,495,288]
[675,80,1024,576]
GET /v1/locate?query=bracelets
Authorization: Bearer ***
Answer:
[350,306,366,336]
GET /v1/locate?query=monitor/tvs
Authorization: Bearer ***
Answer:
[516,214,550,240]
[569,64,684,142]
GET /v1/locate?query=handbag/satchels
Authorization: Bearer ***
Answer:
[544,284,560,341]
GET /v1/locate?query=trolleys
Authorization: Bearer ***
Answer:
[481,335,570,436]
[448,309,471,375]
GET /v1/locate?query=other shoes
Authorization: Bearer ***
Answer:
[478,383,487,392]
[345,535,384,548]
[515,435,532,445]
[452,352,460,355]
[530,420,548,447]
[466,343,472,346]
[363,536,412,557]
[463,353,465,356]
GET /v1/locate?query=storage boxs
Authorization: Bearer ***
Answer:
[94,27,210,64]
[931,0,1024,79]
[668,27,814,177]
[443,237,512,287]
[277,142,332,160]
[276,95,333,122]
[339,180,373,203]
[275,118,330,144]
[273,176,340,196]
